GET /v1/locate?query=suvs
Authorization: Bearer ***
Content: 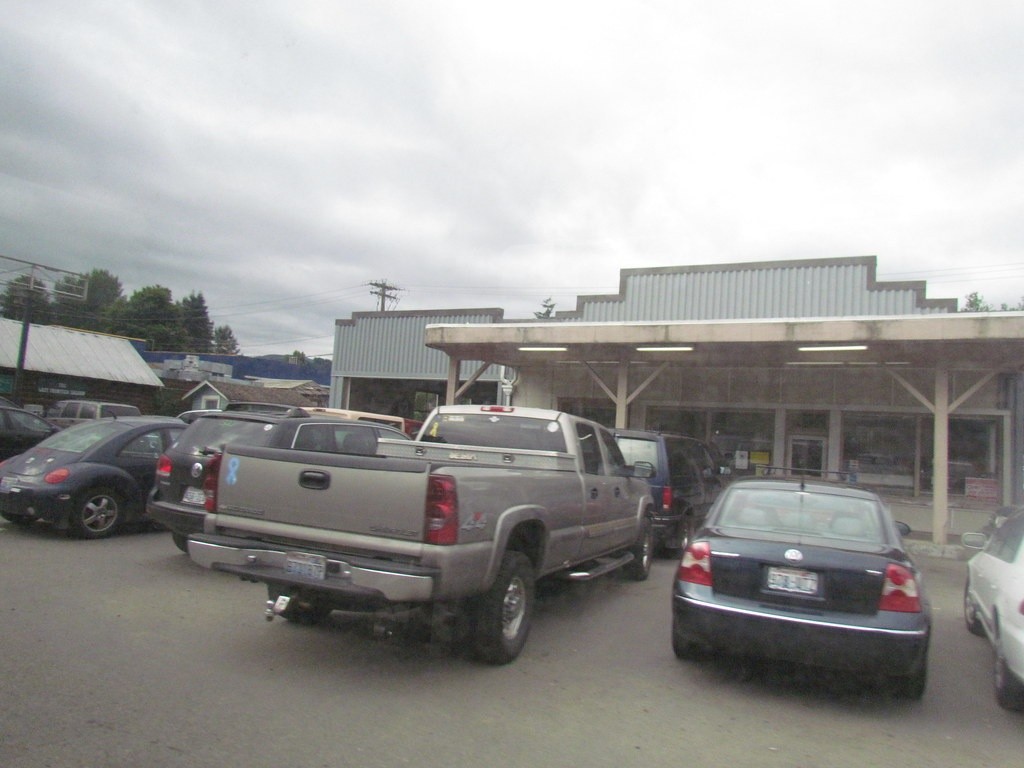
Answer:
[606,426,735,558]
[146,403,413,555]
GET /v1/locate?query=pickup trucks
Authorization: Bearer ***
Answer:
[187,405,657,666]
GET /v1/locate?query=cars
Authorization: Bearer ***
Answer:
[959,505,1024,714]
[671,475,933,698]
[0,394,425,462]
[0,417,190,540]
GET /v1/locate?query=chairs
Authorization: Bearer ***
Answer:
[341,434,373,455]
[826,510,866,538]
[734,507,767,529]
